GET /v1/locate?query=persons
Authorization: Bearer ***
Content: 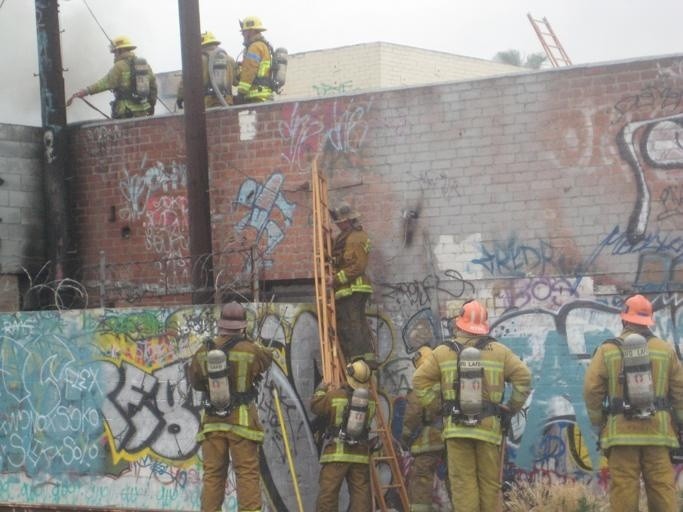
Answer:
[176,31,241,111]
[65,36,157,120]
[322,202,380,378]
[188,301,273,512]
[308,356,380,512]
[233,15,277,103]
[401,347,454,512]
[584,295,683,512]
[408,299,532,512]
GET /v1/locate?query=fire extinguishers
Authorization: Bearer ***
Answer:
[623,334,655,419]
[132,57,150,104]
[204,349,229,415]
[212,51,227,98]
[345,388,368,446]
[459,347,481,426]
[273,47,287,94]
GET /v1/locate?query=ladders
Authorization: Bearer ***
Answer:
[527,12,574,67]
[311,161,412,511]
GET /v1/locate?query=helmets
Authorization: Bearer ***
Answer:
[201,31,221,46]
[331,201,361,224]
[621,295,657,325]
[239,15,267,31]
[218,302,248,329]
[414,345,432,369]
[456,300,489,334]
[345,359,371,390]
[109,35,137,53]
